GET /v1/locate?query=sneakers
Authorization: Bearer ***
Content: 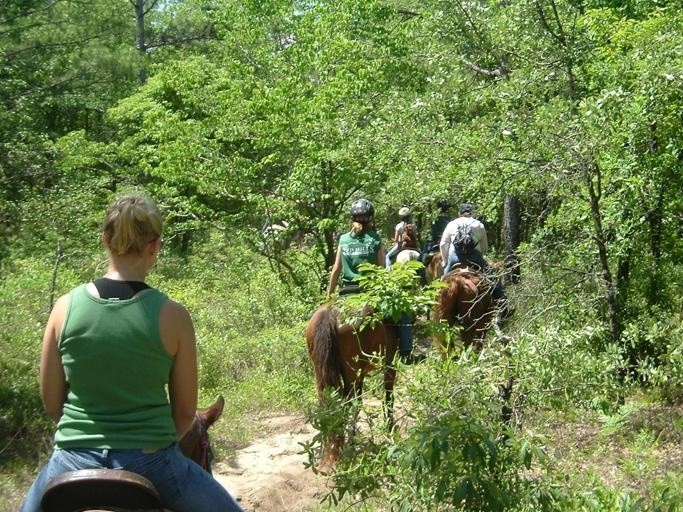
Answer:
[402,352,425,364]
[500,308,515,317]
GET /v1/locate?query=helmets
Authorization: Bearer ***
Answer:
[350,198,374,223]
[438,199,451,208]
[460,202,474,215]
[398,208,411,219]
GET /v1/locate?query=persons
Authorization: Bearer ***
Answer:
[385,208,423,273]
[17,197,242,512]
[439,204,505,299]
[328,199,425,364]
[425,201,450,251]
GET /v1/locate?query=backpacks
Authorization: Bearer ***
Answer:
[401,223,417,246]
[452,218,478,256]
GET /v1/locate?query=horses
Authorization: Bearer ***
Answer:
[176,395,226,477]
[305,246,400,468]
[394,245,515,365]
